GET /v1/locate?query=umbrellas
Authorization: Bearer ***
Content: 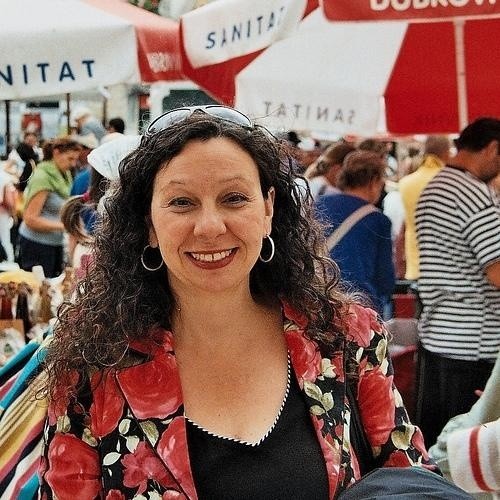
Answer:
[180,0,500,137]
[1,1,179,100]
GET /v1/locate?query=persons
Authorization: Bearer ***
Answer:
[0,113,142,286]
[36,107,445,499]
[286,132,449,325]
[428,356,499,494]
[416,115,499,450]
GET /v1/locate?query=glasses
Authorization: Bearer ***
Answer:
[145,104,254,136]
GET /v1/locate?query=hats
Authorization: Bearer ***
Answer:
[287,131,315,151]
[74,109,89,122]
